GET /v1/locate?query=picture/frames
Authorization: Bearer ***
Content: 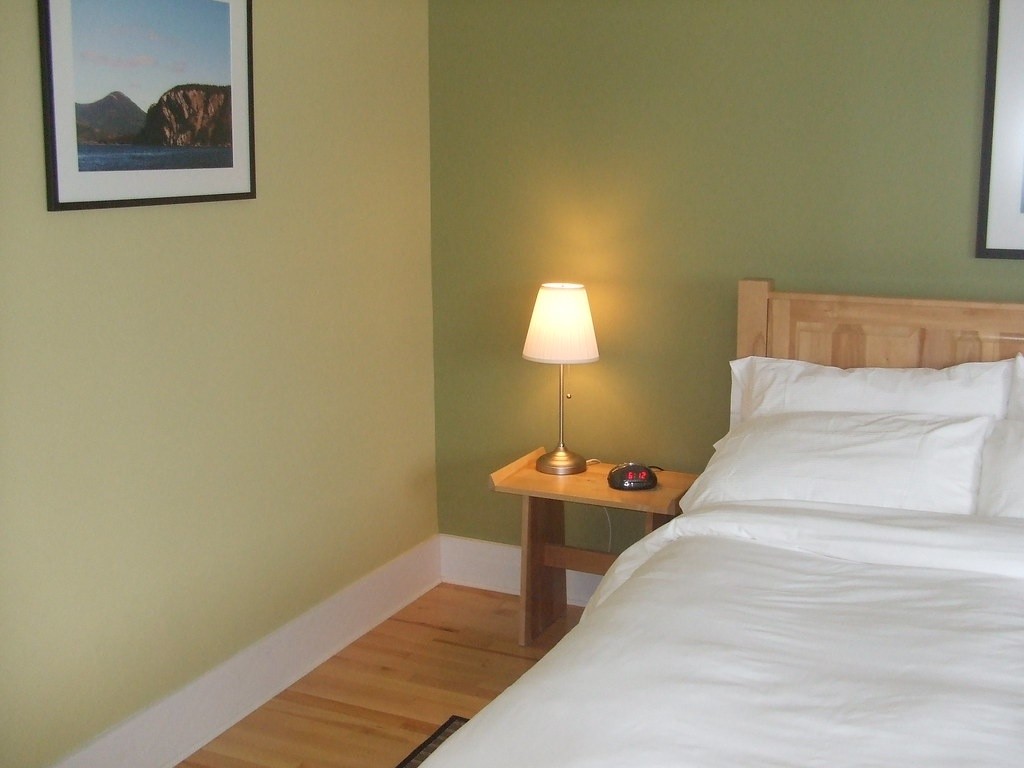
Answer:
[976,1,1024,260]
[38,0,256,212]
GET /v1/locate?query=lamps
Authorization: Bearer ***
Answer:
[522,281,600,476]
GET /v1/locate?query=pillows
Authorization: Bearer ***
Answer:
[1007,352,1024,420]
[979,420,1024,517]
[725,353,1014,422]
[674,415,995,513]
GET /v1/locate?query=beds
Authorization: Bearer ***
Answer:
[413,279,1024,768]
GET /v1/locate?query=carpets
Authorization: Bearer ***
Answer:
[396,714,470,768]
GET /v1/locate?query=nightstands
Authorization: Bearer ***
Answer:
[488,446,700,647]
